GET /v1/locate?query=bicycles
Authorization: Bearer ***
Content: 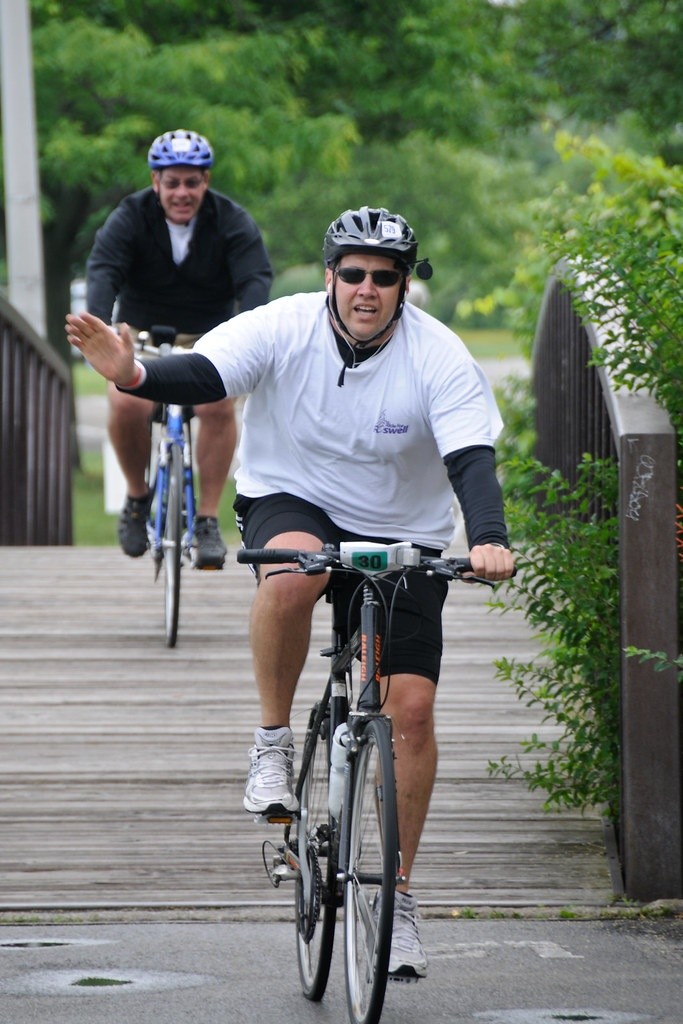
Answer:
[132,332,223,649]
[236,540,518,1024]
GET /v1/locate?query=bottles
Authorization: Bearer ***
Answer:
[326,723,350,821]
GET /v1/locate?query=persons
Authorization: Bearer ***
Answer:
[85,130,274,563]
[62,205,514,980]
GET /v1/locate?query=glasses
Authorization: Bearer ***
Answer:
[325,265,404,287]
[157,177,205,188]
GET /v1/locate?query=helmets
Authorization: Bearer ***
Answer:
[147,129,212,170]
[322,205,417,274]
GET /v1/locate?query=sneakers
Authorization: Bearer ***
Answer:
[115,482,151,556]
[183,516,226,567]
[373,890,430,975]
[243,723,300,815]
